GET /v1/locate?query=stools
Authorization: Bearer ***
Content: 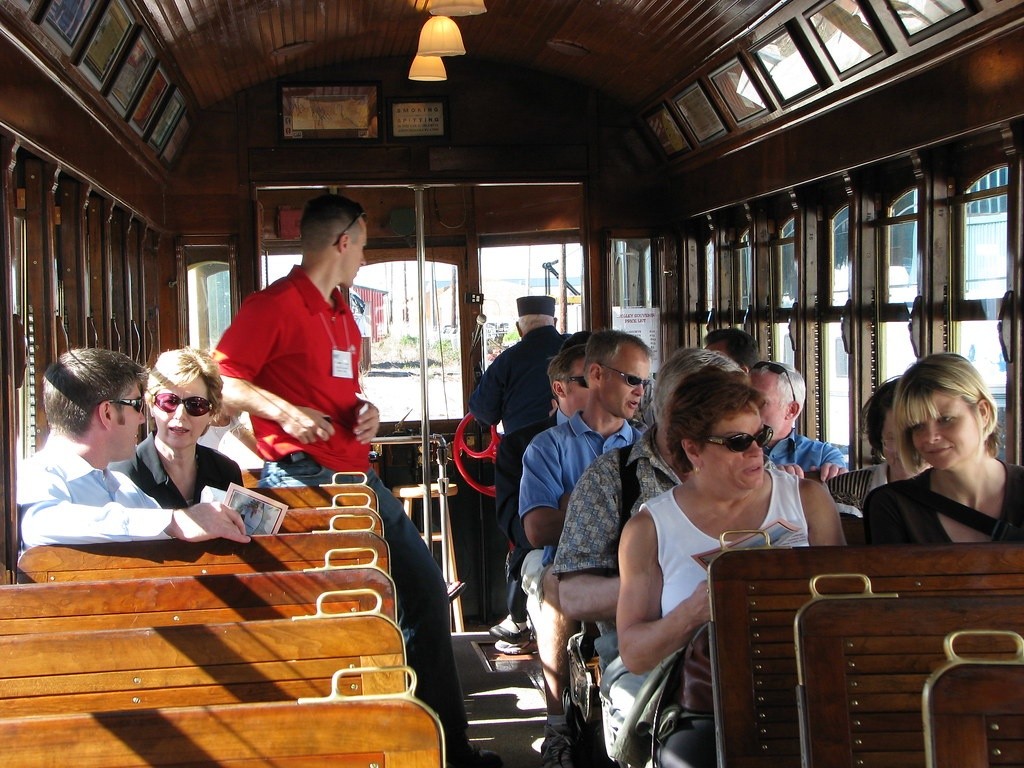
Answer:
[392,482,465,634]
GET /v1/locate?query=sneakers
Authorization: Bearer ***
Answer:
[495,631,537,653]
[540,725,572,768]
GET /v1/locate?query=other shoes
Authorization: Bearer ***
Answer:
[490,622,532,643]
[445,740,503,768]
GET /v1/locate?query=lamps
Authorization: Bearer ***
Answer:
[408,1,488,81]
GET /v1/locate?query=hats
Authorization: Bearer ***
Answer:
[516,296,555,317]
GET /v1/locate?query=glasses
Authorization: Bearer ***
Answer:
[598,364,650,389]
[564,376,590,388]
[753,361,795,402]
[702,423,774,453]
[99,397,143,413]
[152,393,213,416]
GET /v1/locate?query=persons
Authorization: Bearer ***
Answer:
[104,345,245,511]
[832,352,1024,544]
[16,347,251,547]
[207,194,501,768]
[468,295,568,436]
[617,365,846,768]
[697,329,849,483]
[490,330,654,768]
[551,344,751,636]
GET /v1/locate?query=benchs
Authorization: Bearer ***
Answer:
[568,529,1024,768]
[0,471,447,768]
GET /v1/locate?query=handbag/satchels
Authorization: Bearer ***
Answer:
[680,619,717,716]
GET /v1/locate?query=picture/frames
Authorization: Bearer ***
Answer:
[8,0,195,173]
[643,1,981,159]
